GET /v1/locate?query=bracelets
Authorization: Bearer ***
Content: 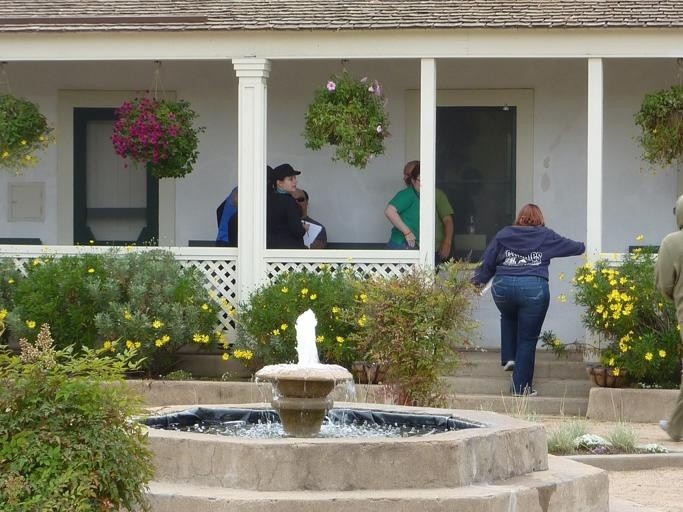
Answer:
[403,231,411,237]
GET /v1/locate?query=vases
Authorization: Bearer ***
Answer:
[664,109,682,133]
[586,367,630,388]
[349,359,393,384]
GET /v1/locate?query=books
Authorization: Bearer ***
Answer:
[300,218,323,248]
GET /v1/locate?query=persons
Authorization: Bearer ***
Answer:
[402,160,456,275]
[471,204,586,397]
[213,164,273,352]
[288,188,327,253]
[266,161,306,285]
[383,166,419,274]
[652,193,682,443]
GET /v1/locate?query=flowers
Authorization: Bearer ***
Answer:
[299,69,394,171]
[110,90,207,182]
[0,92,60,180]
[630,83,683,177]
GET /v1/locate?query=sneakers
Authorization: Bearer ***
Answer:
[504,360,515,371]
[528,389,537,397]
[659,420,680,441]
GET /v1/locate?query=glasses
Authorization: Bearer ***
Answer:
[296,197,305,202]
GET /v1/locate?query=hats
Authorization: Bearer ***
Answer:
[271,164,301,180]
